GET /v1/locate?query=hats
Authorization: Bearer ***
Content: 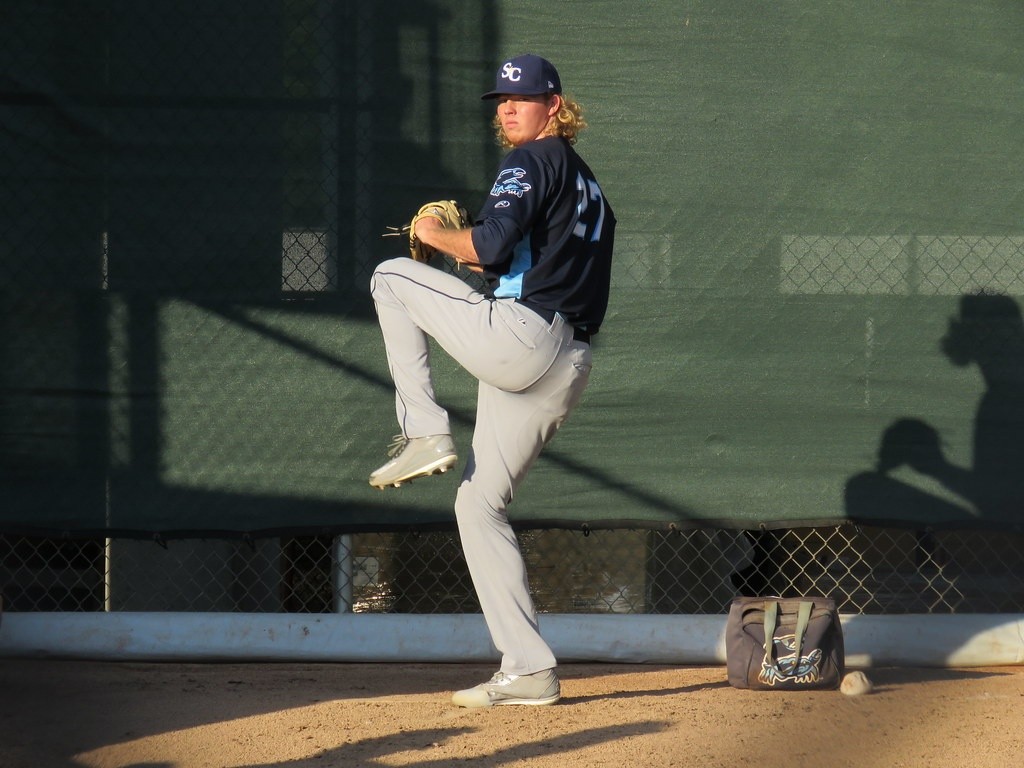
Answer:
[481,54,562,99]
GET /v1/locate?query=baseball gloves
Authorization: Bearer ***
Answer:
[408,199,474,273]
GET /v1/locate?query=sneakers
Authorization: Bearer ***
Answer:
[451,668,560,706]
[368,435,459,489]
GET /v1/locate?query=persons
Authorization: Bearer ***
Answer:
[368,55,617,708]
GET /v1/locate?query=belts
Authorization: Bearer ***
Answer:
[515,298,591,346]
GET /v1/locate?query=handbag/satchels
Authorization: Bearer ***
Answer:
[726,597,844,690]
